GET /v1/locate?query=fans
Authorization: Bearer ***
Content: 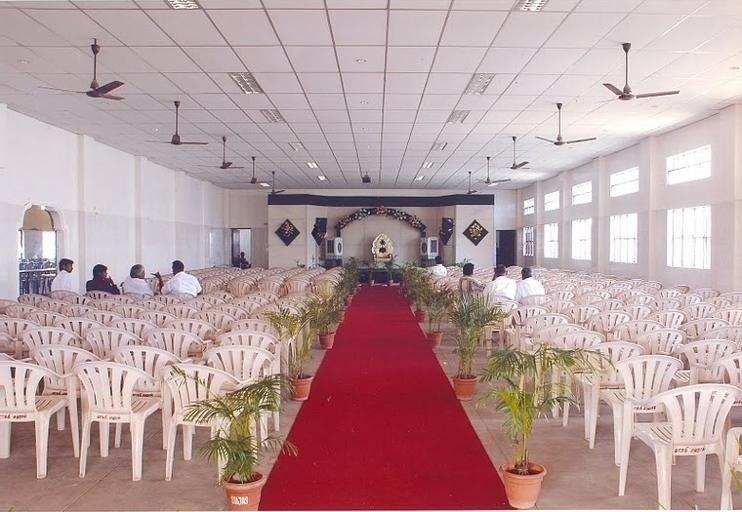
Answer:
[460,38,693,200]
[38,37,124,101]
[257,171,285,194]
[240,156,271,184]
[144,101,209,145]
[192,135,245,169]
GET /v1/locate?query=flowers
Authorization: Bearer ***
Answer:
[334,205,428,234]
[470,222,483,241]
[279,220,295,239]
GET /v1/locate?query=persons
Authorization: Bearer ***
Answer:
[51,259,79,295]
[240,251,249,264]
[427,256,447,277]
[481,265,516,315]
[86,264,121,295]
[123,264,154,298]
[459,263,484,293]
[151,261,204,297]
[515,267,545,303]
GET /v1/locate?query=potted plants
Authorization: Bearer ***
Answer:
[169,362,298,510]
[446,290,512,401]
[382,254,399,286]
[368,261,376,286]
[262,257,359,397]
[472,343,616,509]
[401,260,459,348]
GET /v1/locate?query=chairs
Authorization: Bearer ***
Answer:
[589,350,742,511]
[519,279,742,442]
[417,265,624,355]
[0,290,310,482]
[144,264,347,303]
